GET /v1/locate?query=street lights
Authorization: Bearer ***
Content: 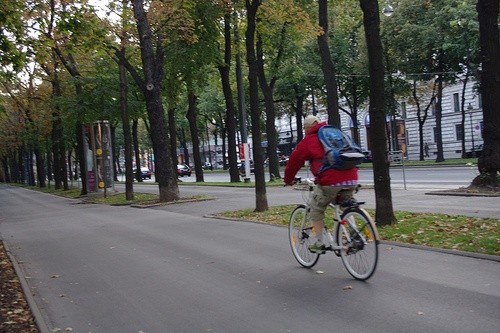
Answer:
[467,103,476,158]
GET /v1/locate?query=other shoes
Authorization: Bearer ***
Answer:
[342,229,363,240]
[310,241,326,254]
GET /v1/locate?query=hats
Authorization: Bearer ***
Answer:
[302,115,320,129]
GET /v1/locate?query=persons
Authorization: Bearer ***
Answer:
[284,115,359,254]
[425,142,430,158]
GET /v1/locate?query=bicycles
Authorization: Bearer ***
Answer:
[282,177,380,281]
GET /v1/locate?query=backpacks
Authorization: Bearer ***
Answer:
[317,125,365,175]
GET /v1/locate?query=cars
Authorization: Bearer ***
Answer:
[132,167,151,181]
[67,171,79,180]
[237,159,255,176]
[176,164,192,178]
[201,162,213,170]
